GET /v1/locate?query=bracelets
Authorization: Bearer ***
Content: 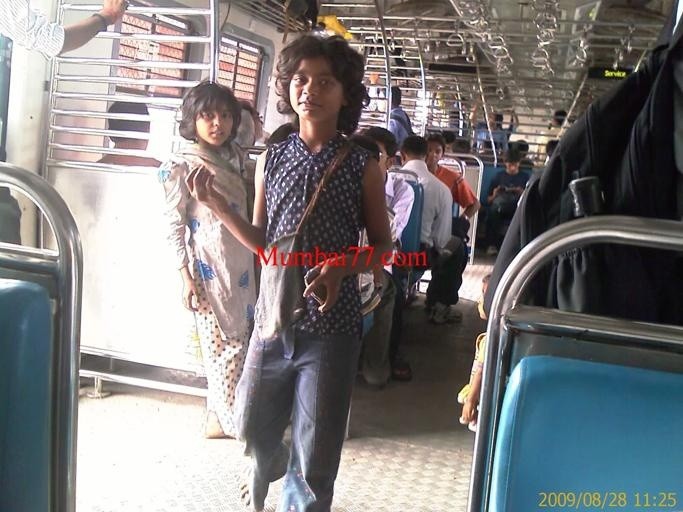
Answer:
[92,12,108,33]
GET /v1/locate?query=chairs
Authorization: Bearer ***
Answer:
[388,156,465,307]
[467,214,682,512]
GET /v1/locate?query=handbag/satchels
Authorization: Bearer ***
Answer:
[254,233,307,344]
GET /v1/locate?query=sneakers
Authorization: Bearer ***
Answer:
[391,347,413,381]
[428,302,463,324]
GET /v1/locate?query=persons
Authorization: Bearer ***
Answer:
[97,101,162,168]
[0,0,129,246]
[160,13,683,511]
